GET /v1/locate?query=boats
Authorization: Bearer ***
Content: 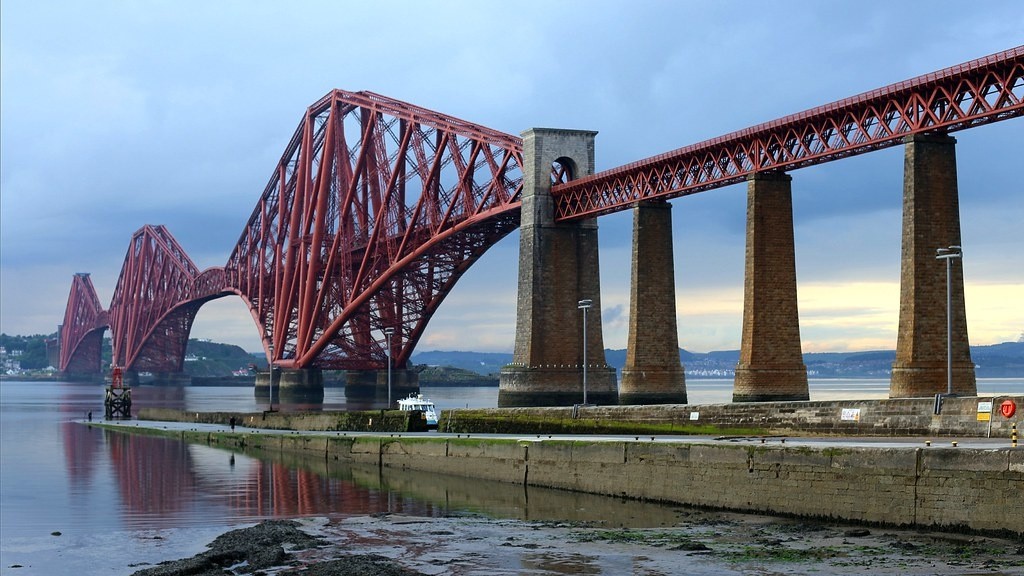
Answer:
[396,394,438,425]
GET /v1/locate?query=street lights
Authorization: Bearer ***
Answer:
[936,245,964,395]
[386,327,394,408]
[268,342,274,411]
[577,299,591,404]
